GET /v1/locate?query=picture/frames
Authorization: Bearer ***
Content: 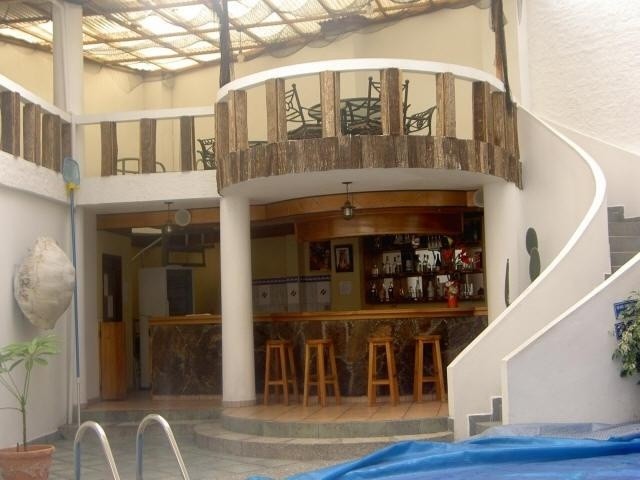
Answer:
[335,245,353,273]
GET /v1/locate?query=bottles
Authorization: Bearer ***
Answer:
[369,235,474,302]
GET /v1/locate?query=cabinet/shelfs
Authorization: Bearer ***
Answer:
[364,217,483,304]
[138,265,195,389]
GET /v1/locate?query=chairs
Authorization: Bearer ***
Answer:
[197,139,216,170]
[278,77,437,141]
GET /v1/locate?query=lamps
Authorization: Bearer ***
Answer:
[341,181,356,220]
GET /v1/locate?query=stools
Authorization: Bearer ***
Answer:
[263,335,446,406]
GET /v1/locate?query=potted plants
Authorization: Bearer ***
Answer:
[0,334,65,480]
